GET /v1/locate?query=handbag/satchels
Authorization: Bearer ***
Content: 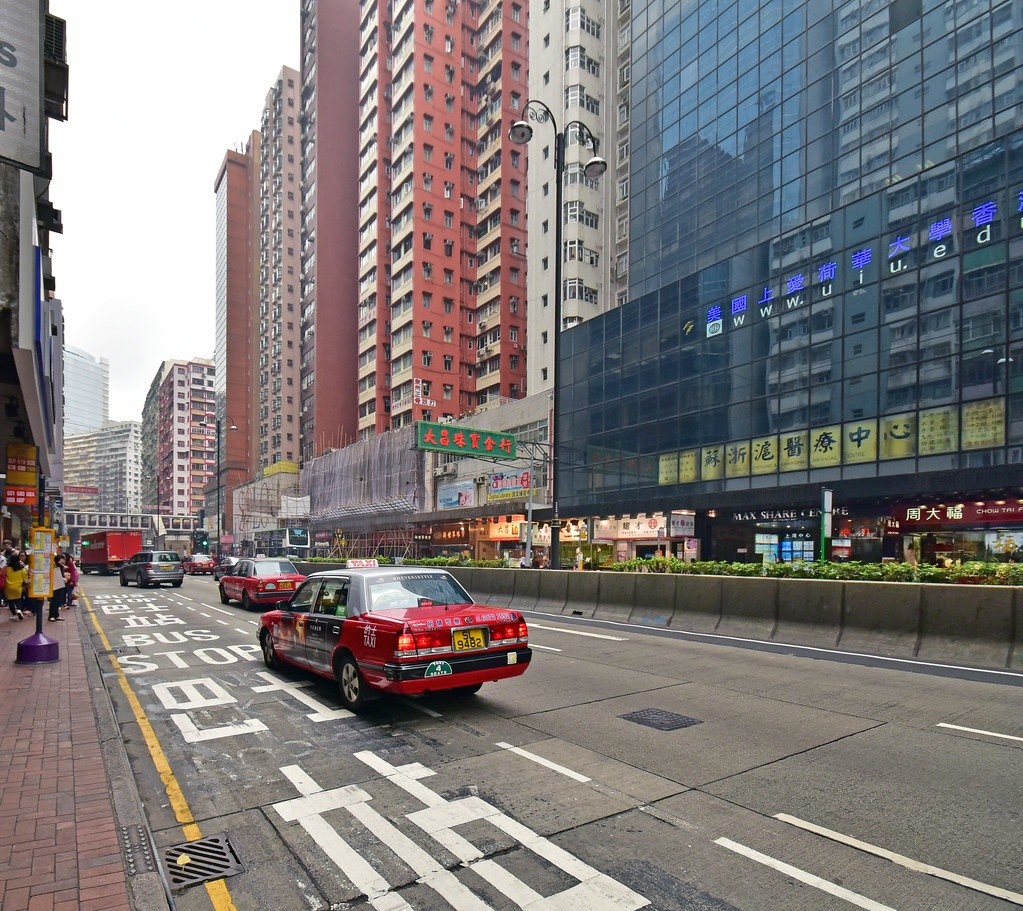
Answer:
[67,594,77,600]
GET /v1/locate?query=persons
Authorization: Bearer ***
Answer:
[17,551,28,568]
[23,554,37,616]
[456,489,473,506]
[61,552,79,611]
[0,540,20,557]
[3,555,29,623]
[69,556,77,570]
[46,555,66,623]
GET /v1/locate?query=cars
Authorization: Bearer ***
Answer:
[217,557,309,610]
[213,557,244,581]
[181,554,217,576]
[257,559,534,709]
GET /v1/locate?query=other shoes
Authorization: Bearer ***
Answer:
[49,616,65,622]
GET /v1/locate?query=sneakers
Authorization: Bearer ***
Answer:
[10,610,23,621]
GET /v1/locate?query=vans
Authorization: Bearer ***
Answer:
[119,549,184,589]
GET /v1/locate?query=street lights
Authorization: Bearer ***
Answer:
[200,413,238,560]
[147,471,170,551]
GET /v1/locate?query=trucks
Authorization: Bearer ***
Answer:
[79,529,143,575]
